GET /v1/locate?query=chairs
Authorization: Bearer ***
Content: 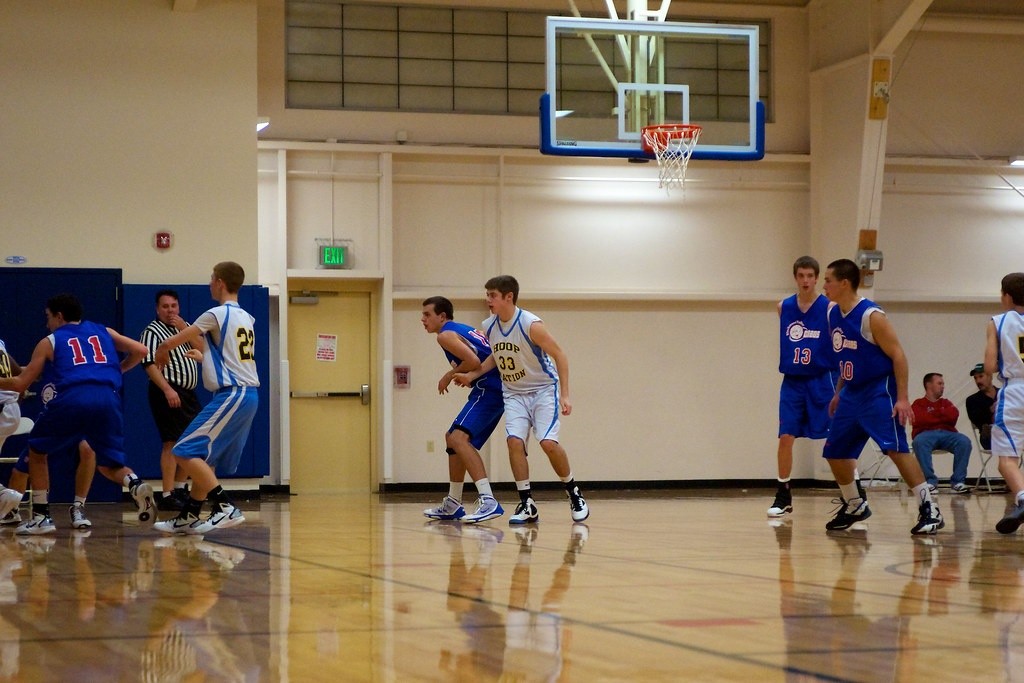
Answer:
[932,450,953,489]
[0,417,33,523]
[971,421,1024,492]
[858,436,914,490]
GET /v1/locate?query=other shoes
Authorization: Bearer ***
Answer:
[160,482,192,510]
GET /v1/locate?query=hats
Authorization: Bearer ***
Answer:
[970,364,983,376]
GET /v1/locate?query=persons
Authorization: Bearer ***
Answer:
[984,272,1024,534]
[452,275,590,526]
[421,297,506,524]
[0,292,159,536]
[907,372,974,493]
[0,339,24,521]
[137,261,261,535]
[768,257,868,518]
[967,363,1000,450]
[819,260,943,535]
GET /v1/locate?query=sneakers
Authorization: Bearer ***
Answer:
[767,489,793,517]
[424,496,467,521]
[508,525,537,545]
[826,496,872,531]
[69,530,92,551]
[462,496,504,523]
[15,514,56,535]
[154,535,204,551]
[195,542,245,569]
[926,485,938,493]
[69,504,91,529]
[135,541,154,591]
[17,536,55,555]
[911,535,942,566]
[827,532,870,556]
[129,479,157,525]
[462,524,503,549]
[564,523,589,565]
[509,498,539,524]
[951,483,971,493]
[424,521,461,539]
[769,520,792,532]
[194,503,245,533]
[154,512,203,535]
[996,491,1024,534]
[910,488,946,534]
[566,486,589,522]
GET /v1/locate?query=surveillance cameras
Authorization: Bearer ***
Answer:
[396,131,408,145]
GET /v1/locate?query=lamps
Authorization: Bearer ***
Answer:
[257,116,270,132]
[1009,155,1024,166]
